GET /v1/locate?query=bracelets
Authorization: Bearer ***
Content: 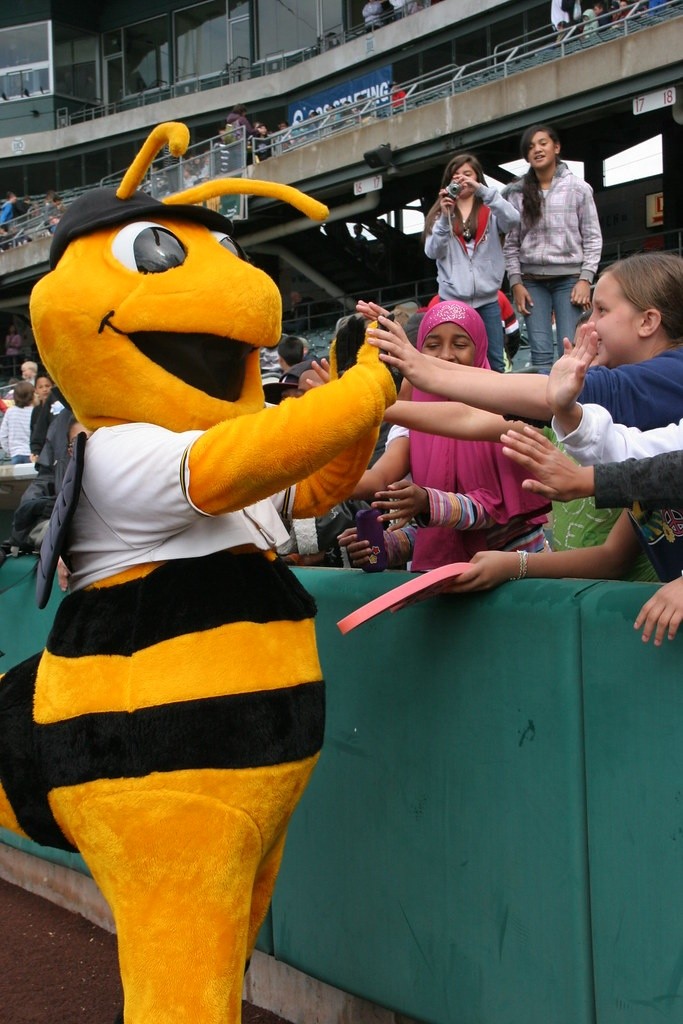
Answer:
[510,550,528,581]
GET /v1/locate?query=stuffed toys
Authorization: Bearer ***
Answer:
[0,122,405,1024]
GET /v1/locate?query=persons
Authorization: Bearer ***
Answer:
[248,95,371,166]
[226,103,267,166]
[0,361,91,593]
[182,129,230,189]
[4,325,22,355]
[503,125,603,366]
[0,190,65,253]
[424,154,520,374]
[388,81,407,114]
[356,252,683,648]
[555,0,667,49]
[259,301,420,572]
[362,0,431,34]
[337,300,552,574]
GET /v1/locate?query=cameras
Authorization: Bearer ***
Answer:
[443,180,463,201]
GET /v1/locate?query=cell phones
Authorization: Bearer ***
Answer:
[356,509,385,573]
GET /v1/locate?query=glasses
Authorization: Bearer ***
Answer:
[460,223,472,243]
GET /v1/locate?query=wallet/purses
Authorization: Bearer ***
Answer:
[356,507,387,573]
